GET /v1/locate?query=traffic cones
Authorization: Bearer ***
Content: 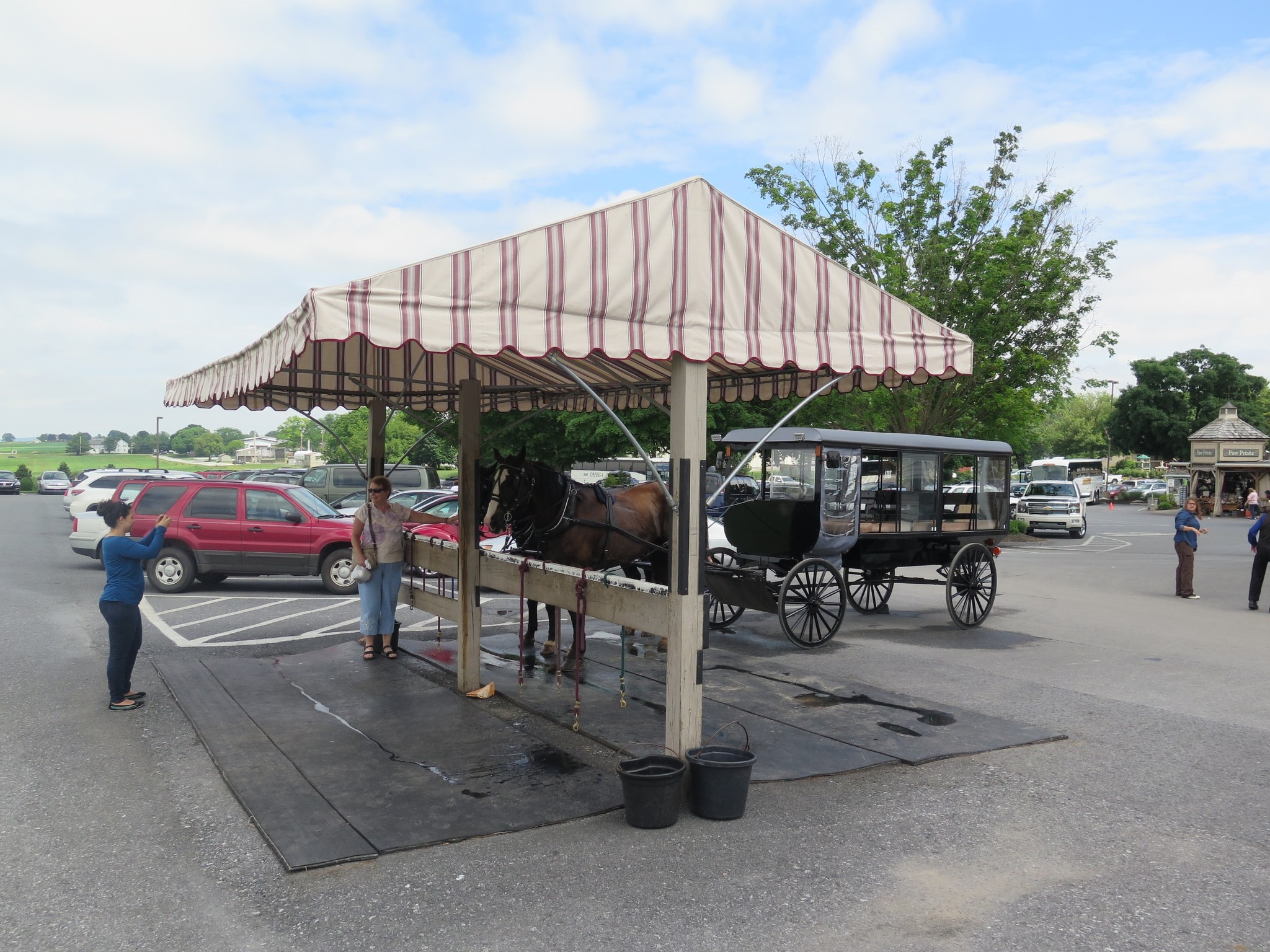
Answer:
[1109,502,1114,510]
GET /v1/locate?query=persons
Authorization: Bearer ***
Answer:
[1174,497,1209,600]
[349,476,459,660]
[1244,488,1260,520]
[1247,500,1270,613]
[96,499,172,713]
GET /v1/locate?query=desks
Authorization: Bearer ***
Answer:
[1202,502,1238,511]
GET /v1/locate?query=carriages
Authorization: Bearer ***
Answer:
[482,429,1012,672]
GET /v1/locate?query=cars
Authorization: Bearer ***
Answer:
[37,471,74,495]
[1126,482,1166,501]
[0,470,23,495]
[1121,478,1165,488]
[479,516,739,583]
[1103,484,1135,501]
[569,456,1032,545]
[153,449,179,456]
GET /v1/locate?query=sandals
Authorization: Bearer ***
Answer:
[363,645,375,659]
[381,646,397,659]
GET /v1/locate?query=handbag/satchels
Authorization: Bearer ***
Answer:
[1246,507,1251,516]
[349,545,378,572]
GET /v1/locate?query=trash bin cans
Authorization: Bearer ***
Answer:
[1149,498,1157,510]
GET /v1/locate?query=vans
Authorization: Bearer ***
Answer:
[277,463,442,504]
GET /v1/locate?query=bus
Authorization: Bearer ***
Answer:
[1031,456,1103,506]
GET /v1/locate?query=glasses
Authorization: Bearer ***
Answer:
[120,501,128,516]
[1188,502,1196,506]
[368,488,385,493]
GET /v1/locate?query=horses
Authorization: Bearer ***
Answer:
[457,447,709,672]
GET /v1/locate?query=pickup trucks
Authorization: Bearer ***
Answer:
[1102,471,1123,485]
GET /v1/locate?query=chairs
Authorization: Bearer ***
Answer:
[1260,498,1269,512]
[1063,490,1072,495]
[246,496,256,519]
[256,497,271,518]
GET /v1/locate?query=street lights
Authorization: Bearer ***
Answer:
[1106,380,1120,490]
[156,416,163,469]
[321,430,325,443]
[299,427,306,451]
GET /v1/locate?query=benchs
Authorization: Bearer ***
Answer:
[825,489,1005,532]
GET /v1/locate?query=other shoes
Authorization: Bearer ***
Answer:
[125,692,146,699]
[1250,518,1257,520]
[1249,600,1259,609]
[1185,594,1200,599]
[109,700,144,711]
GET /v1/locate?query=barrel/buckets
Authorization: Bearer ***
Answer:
[615,741,686,830]
[374,618,402,646]
[685,720,758,821]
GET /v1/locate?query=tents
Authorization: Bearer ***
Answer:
[162,178,976,759]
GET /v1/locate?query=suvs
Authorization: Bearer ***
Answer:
[232,460,246,465]
[1014,480,1091,539]
[133,478,363,596]
[63,466,515,577]
